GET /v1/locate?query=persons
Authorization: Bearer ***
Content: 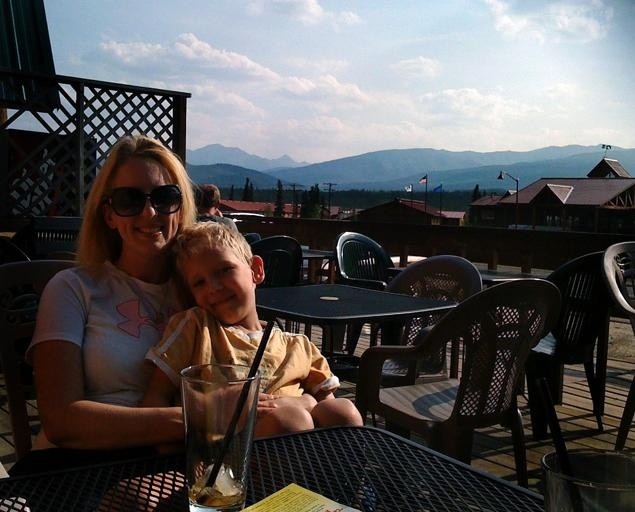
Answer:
[20,136,287,449]
[195,184,239,232]
[136,221,364,436]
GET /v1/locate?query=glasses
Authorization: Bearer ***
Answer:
[105,182,183,219]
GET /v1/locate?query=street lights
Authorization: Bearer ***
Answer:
[497,169,519,229]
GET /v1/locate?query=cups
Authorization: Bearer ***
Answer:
[178,364,260,512]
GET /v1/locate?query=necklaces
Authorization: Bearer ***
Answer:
[119,271,175,326]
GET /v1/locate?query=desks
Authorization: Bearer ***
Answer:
[252,282,460,363]
[0,420,548,510]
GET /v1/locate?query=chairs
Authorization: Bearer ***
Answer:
[1,258,93,474]
[6,233,482,374]
[525,246,606,436]
[355,276,559,494]
[603,239,635,452]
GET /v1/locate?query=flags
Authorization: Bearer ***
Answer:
[405,184,413,193]
[434,185,443,191]
[420,176,426,184]
